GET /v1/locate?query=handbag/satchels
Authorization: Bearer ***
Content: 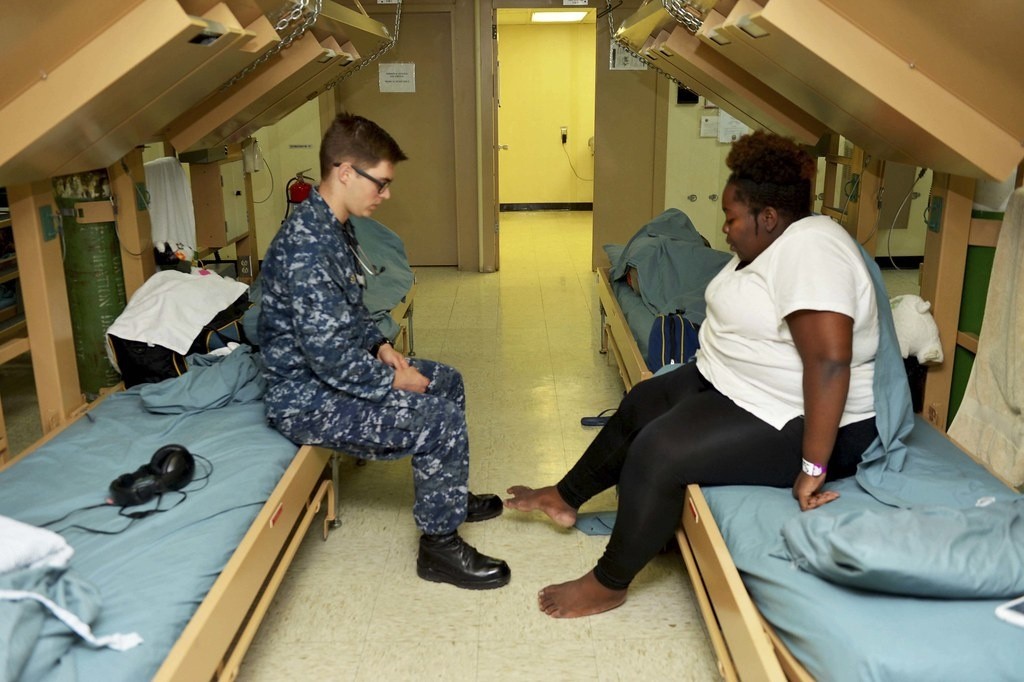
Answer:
[647,308,700,372]
[107,292,258,391]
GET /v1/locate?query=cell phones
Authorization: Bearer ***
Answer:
[996,596,1024,627]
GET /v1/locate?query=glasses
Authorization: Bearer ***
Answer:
[333,162,390,194]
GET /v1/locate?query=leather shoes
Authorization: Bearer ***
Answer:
[417,529,511,590]
[465,491,504,522]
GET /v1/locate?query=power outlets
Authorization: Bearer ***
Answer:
[560,127,568,139]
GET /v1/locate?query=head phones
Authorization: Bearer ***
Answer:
[109,444,195,507]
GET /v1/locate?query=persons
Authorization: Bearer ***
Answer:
[504,128,912,619]
[256,109,510,591]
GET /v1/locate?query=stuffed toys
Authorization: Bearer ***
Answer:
[890,294,944,365]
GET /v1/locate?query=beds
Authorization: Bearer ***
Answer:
[594,267,1024,682]
[0,267,419,682]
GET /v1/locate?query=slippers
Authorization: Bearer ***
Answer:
[581,408,618,426]
[623,390,626,396]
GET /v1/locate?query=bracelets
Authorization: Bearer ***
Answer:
[802,457,827,476]
